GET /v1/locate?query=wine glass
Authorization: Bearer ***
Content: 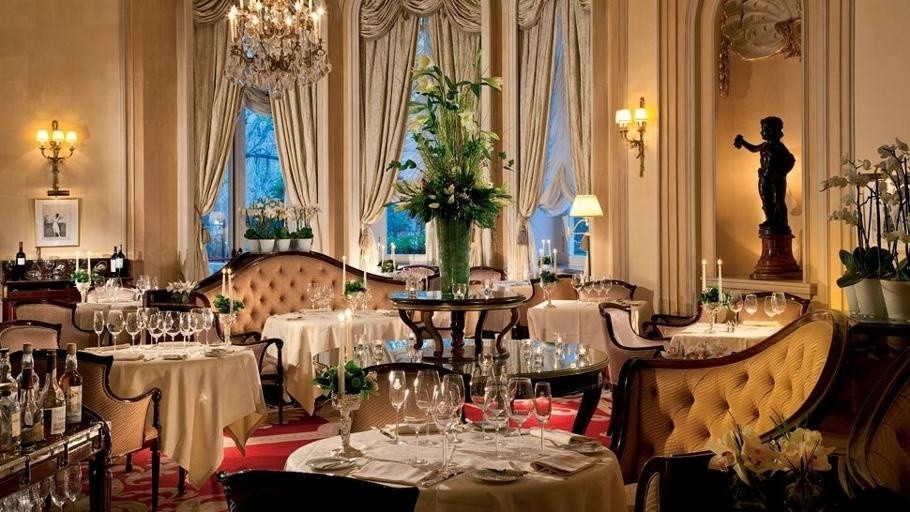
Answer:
[402,267,531,301]
[0,462,85,512]
[540,274,558,309]
[382,371,553,483]
[73,271,234,363]
[570,273,615,310]
[305,281,373,318]
[701,286,789,334]
[4,240,106,282]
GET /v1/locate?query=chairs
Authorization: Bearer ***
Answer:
[1,319,63,354]
[649,284,810,342]
[73,247,645,434]
[11,297,98,372]
[597,300,666,457]
[617,305,850,511]
[54,347,188,512]
[215,471,421,512]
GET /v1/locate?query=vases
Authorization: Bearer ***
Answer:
[841,276,905,323]
[437,212,469,297]
[249,239,313,254]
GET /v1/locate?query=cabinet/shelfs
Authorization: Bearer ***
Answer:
[1,347,112,511]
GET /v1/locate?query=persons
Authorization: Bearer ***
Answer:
[51,213,62,237]
[734,117,795,231]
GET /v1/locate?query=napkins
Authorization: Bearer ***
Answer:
[351,456,439,486]
[387,418,441,436]
[529,448,602,478]
[528,428,591,449]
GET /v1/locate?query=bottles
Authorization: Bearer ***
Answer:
[110,243,127,278]
[0,338,85,469]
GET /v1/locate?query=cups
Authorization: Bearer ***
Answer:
[348,325,592,371]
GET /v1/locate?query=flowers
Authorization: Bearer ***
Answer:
[384,53,516,230]
[820,138,910,288]
[241,197,320,239]
[701,415,832,507]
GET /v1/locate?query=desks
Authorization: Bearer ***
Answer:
[3,259,133,329]
[85,340,269,498]
[666,320,784,361]
[827,315,910,511]
[285,426,630,510]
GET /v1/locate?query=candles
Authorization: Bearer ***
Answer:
[719,259,723,305]
[541,239,552,263]
[702,260,707,295]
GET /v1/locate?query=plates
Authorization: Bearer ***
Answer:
[565,443,609,455]
[304,457,357,472]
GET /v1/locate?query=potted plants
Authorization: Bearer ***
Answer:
[701,286,729,333]
[316,360,377,458]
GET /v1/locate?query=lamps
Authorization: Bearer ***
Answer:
[567,194,603,236]
[615,96,646,177]
[220,1,332,95]
[35,119,78,193]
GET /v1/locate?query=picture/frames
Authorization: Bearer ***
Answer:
[34,197,81,247]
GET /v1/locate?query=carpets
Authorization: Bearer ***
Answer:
[119,418,316,510]
